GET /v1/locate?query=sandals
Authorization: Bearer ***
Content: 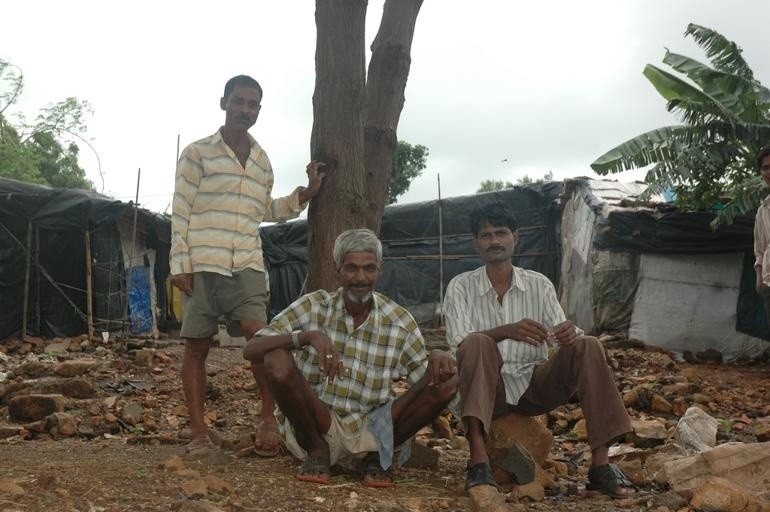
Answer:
[295,456,333,485]
[465,460,501,493]
[347,457,396,488]
[254,420,283,457]
[585,462,641,500]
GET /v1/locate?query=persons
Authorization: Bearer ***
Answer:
[243,229,459,487]
[169,75,326,456]
[443,203,640,499]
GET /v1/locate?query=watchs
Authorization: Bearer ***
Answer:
[292,330,301,349]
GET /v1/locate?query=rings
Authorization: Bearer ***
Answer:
[327,354,333,359]
[338,360,343,364]
